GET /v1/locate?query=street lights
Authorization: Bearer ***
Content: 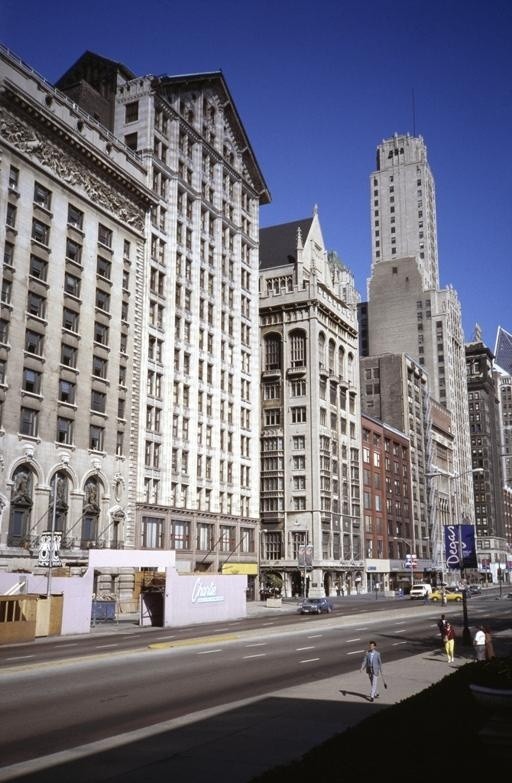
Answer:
[257,528,267,603]
[392,535,430,588]
[293,521,308,599]
[424,467,484,645]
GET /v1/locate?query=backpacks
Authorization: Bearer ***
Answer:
[443,635,449,643]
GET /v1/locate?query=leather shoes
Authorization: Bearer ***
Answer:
[371,693,379,702]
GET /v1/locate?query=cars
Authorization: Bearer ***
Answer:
[410,582,482,602]
[299,597,332,615]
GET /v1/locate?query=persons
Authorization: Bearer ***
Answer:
[437,612,449,648]
[442,621,456,664]
[359,640,387,703]
[471,623,486,662]
[422,590,430,604]
[481,621,496,660]
